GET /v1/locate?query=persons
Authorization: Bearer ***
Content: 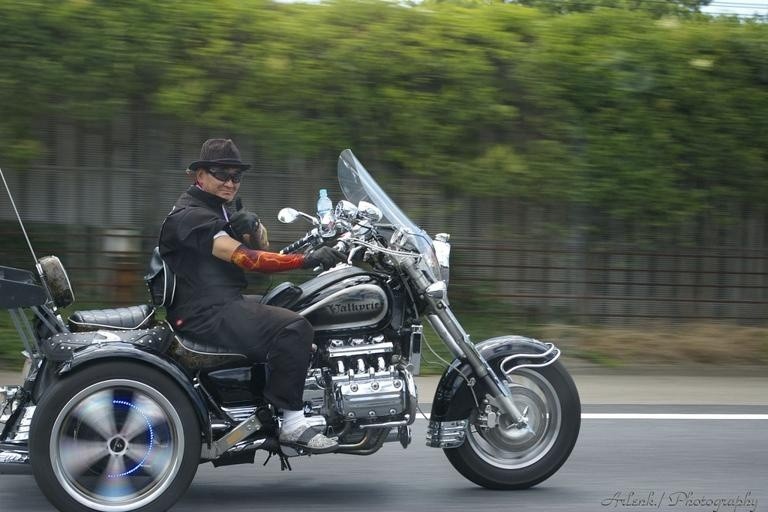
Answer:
[148,133,349,459]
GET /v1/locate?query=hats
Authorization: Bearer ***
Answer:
[188,139,252,172]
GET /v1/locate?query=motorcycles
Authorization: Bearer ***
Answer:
[0,149,581,512]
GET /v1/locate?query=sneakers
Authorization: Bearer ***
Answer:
[279,423,340,454]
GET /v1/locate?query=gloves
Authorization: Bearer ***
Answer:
[303,245,347,271]
[228,196,256,235]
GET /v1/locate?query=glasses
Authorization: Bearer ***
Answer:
[204,168,243,184]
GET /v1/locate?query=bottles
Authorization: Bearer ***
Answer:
[315,188,336,239]
